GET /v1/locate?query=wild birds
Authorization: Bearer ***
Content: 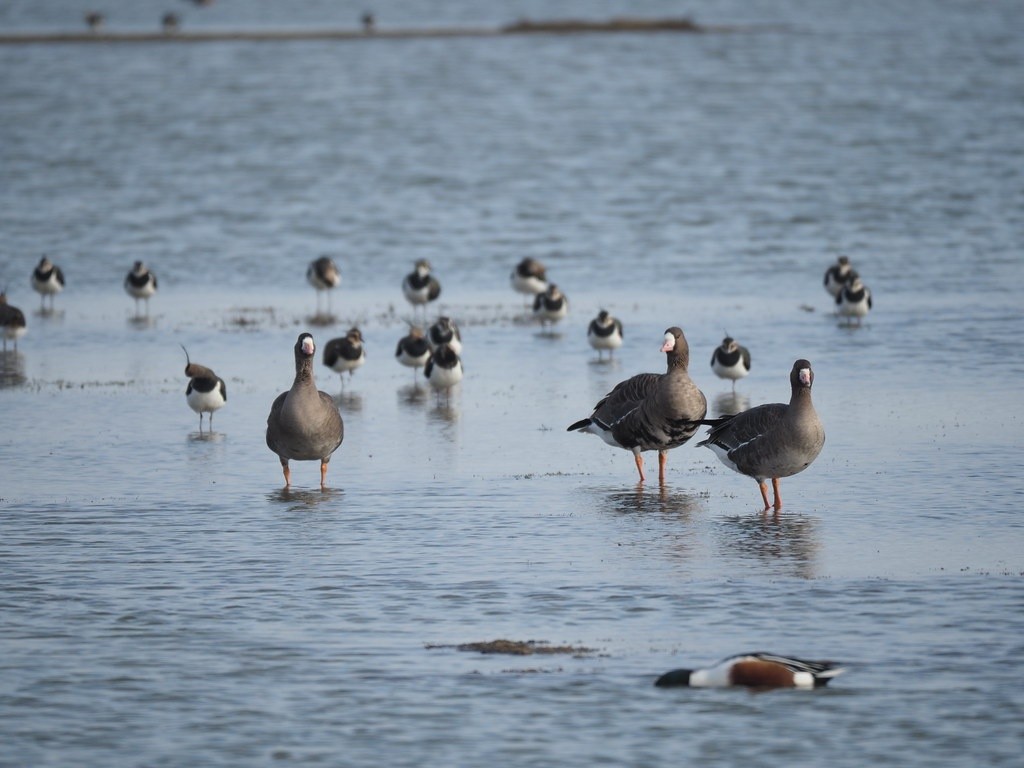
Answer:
[125,259,158,318]
[824,255,872,328]
[178,343,228,440]
[709,336,751,391]
[655,652,852,690]
[31,253,65,308]
[693,359,825,511]
[0,290,27,348]
[567,326,708,480]
[509,256,566,332]
[586,309,624,362]
[307,253,342,306]
[396,314,463,395]
[402,258,441,310]
[265,332,344,490]
